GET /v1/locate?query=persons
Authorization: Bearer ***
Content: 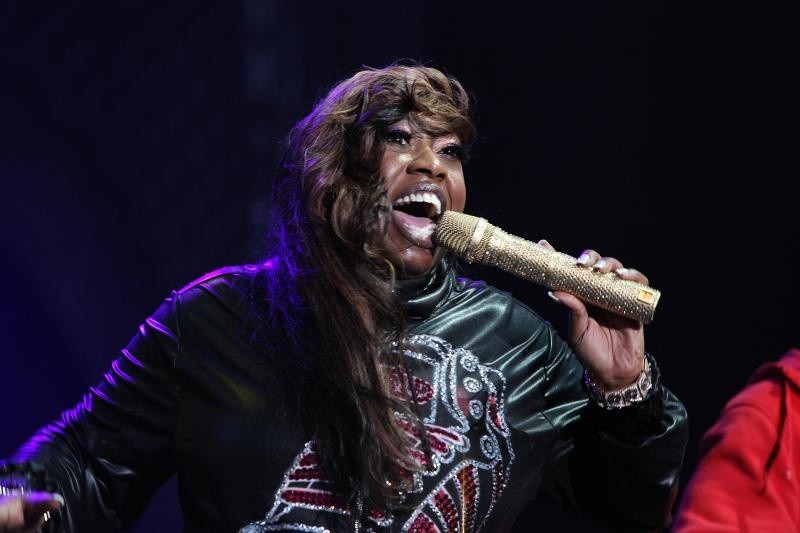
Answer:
[670,347,800,533]
[1,66,688,533]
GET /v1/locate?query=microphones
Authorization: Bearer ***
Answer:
[432,210,662,325]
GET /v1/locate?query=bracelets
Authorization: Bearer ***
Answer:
[584,356,653,409]
